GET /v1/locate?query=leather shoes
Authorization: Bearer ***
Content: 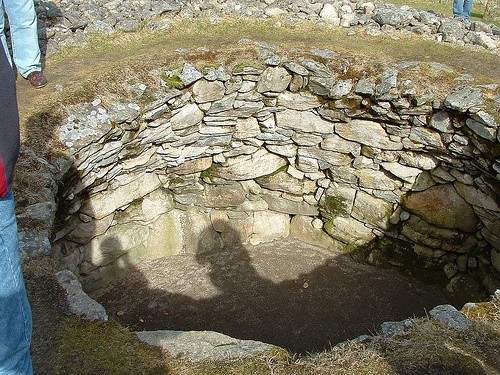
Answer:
[28,71,47,87]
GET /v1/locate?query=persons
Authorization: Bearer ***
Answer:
[453,0,473,21]
[0,0,47,88]
[1,38,34,375]
